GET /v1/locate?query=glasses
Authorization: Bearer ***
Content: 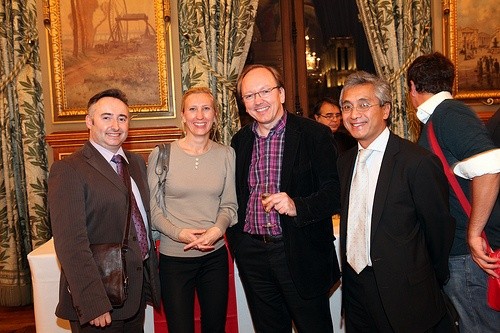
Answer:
[240,86,280,102]
[316,112,342,121]
[339,102,380,111]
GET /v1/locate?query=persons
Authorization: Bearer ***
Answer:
[48,87,149,333]
[224,65,338,333]
[313,97,357,150]
[334,70,460,333]
[406,53,500,333]
[146,86,238,333]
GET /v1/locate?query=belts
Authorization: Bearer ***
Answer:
[242,232,282,244]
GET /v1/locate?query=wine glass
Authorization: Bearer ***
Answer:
[261,183,276,227]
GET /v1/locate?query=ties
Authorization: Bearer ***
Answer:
[110,155,150,262]
[346,149,369,274]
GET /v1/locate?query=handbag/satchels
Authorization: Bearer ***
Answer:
[486,248,500,312]
[151,141,170,248]
[66,243,128,307]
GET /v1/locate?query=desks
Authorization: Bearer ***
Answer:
[26,218,347,333]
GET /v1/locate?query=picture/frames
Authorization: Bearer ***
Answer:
[41,0,177,126]
[440,0,500,107]
